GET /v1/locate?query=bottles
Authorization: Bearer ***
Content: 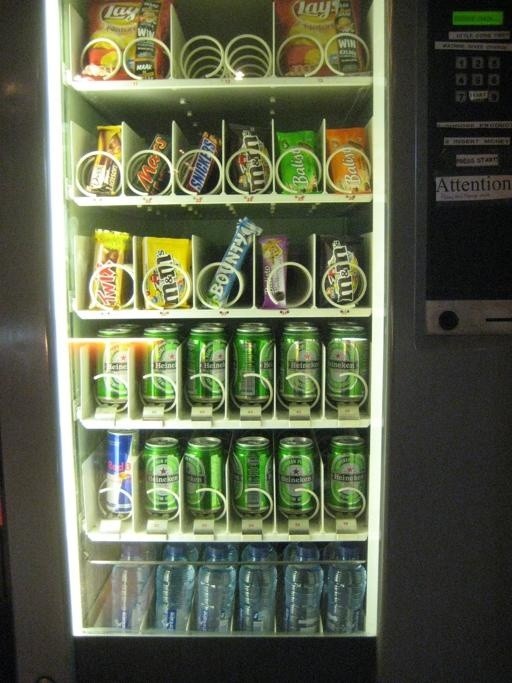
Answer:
[108,544,367,637]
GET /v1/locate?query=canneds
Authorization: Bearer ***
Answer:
[94,321,370,403]
[105,430,367,514]
